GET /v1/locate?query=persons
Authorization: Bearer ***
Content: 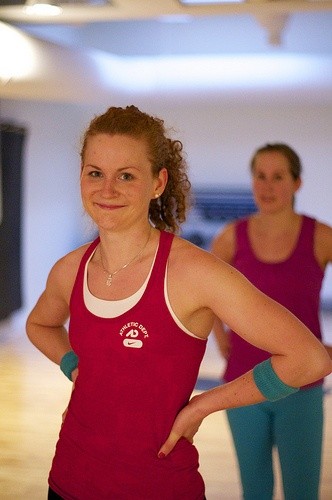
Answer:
[205,143,332,500]
[25,104,332,500]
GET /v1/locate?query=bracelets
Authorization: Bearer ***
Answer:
[59,350,79,382]
[253,357,300,403]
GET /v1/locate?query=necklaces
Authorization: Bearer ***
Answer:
[99,224,152,288]
